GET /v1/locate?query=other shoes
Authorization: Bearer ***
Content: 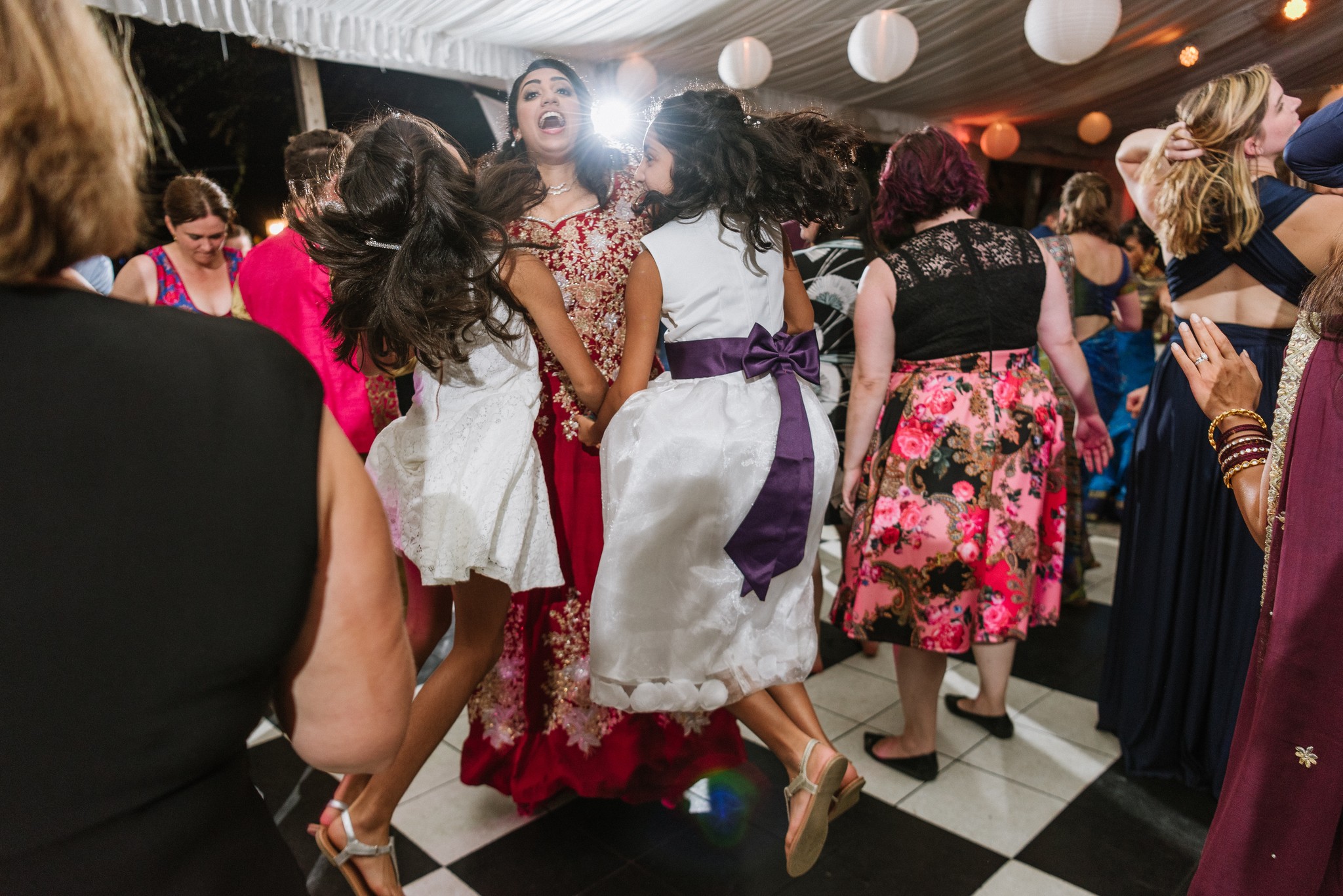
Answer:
[864,731,938,782]
[945,692,1013,737]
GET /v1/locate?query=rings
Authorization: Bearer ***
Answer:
[1193,351,1209,366]
[839,503,847,508]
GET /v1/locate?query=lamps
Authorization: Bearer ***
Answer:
[1178,41,1199,67]
[979,122,1021,159]
[1077,111,1112,144]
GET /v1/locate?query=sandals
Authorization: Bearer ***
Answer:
[316,814,402,896]
[781,739,845,879]
[827,757,865,821]
[307,797,352,838]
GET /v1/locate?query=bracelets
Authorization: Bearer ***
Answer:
[1208,409,1268,452]
[1216,423,1273,488]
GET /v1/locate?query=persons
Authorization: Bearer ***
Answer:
[781,209,880,676]
[1166,257,1342,896]
[223,122,407,454]
[571,85,876,881]
[836,127,1118,785]
[544,173,580,195]
[285,105,609,896]
[1,0,423,896]
[1110,62,1343,821]
[105,170,251,320]
[1282,95,1343,189]
[448,50,749,817]
[1032,166,1143,610]
[1109,216,1172,519]
